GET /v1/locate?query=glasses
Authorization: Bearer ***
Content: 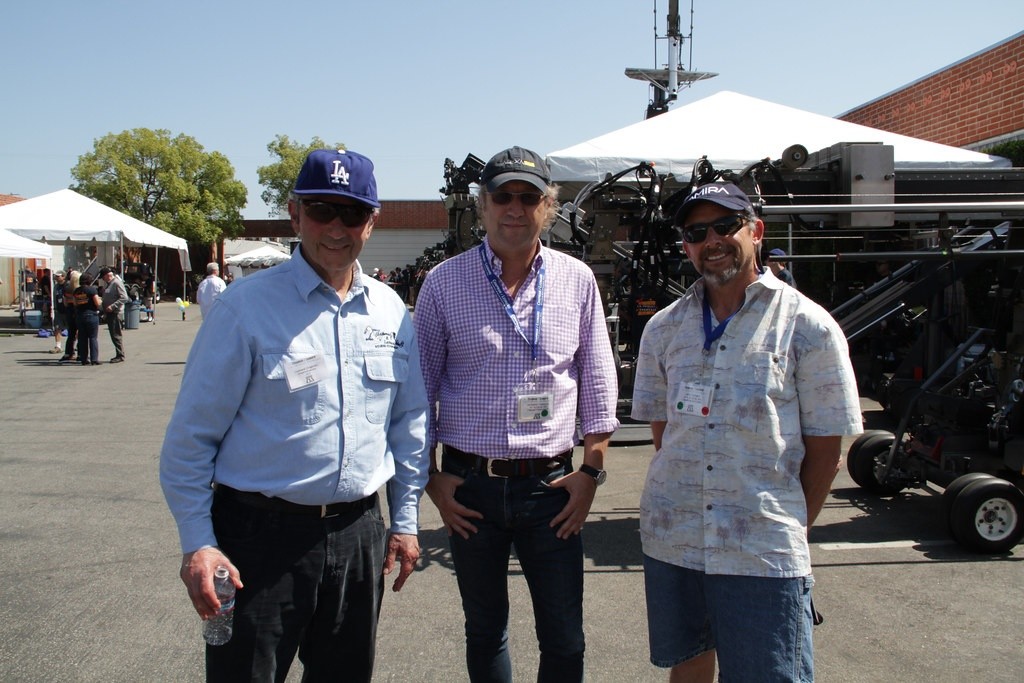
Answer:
[486,191,544,205]
[299,198,375,227]
[682,213,751,243]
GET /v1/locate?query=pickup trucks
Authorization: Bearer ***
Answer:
[123,263,163,304]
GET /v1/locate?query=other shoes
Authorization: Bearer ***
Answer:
[111,357,124,363]
[76,357,81,363]
[91,360,102,365]
[81,361,91,365]
[59,354,76,362]
[49,346,64,354]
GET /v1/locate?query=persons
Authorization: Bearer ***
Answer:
[371,261,434,309]
[159,149,431,683]
[43,262,232,366]
[413,147,620,683]
[766,249,796,290]
[630,180,864,683]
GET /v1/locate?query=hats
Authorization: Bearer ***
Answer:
[98,267,111,279]
[684,183,756,214]
[53,270,66,276]
[769,248,786,256]
[292,149,381,207]
[480,146,552,193]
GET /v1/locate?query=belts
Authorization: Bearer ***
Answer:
[442,444,573,478]
[213,482,376,519]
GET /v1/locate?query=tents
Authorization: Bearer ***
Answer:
[225,246,293,278]
[0,189,191,319]
[544,90,1013,195]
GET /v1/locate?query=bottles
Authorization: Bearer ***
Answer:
[202,564,236,646]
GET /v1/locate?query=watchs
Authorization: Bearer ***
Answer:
[579,464,607,486]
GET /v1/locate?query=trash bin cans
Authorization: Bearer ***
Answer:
[125,302,139,329]
